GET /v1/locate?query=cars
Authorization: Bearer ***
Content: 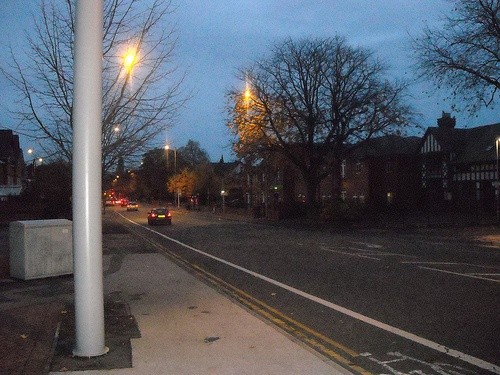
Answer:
[105,192,123,207]
[126,202,139,212]
[121,200,130,207]
[148,207,172,226]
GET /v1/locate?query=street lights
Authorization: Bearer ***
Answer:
[165,145,177,207]
[220,189,227,214]
